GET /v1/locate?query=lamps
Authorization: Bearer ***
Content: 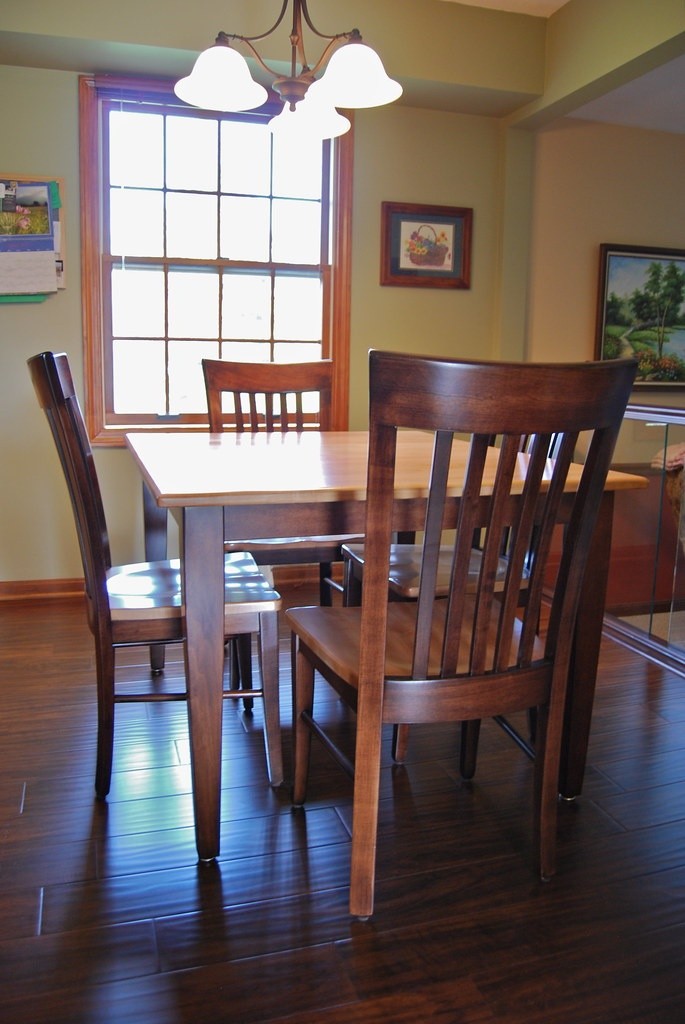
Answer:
[173,0,403,140]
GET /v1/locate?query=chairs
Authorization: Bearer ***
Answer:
[285,347,640,922]
[201,358,364,711]
[339,433,564,780]
[27,352,283,797]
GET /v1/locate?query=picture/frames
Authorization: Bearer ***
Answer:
[380,199,473,290]
[593,242,685,393]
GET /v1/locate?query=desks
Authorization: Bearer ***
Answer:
[123,430,653,862]
